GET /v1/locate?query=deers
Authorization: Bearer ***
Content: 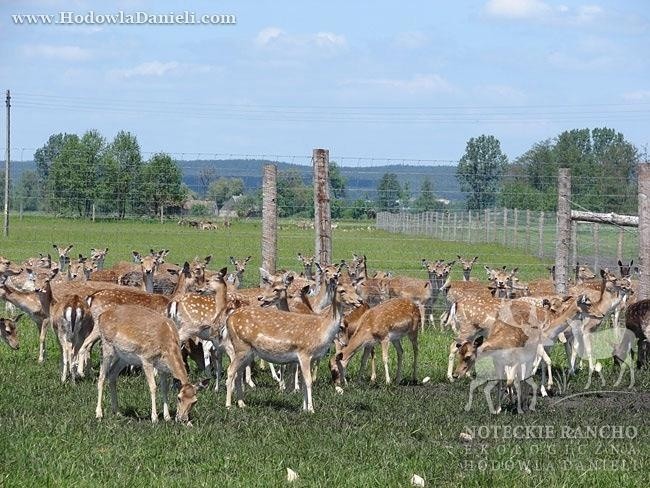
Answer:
[0,242,650,425]
